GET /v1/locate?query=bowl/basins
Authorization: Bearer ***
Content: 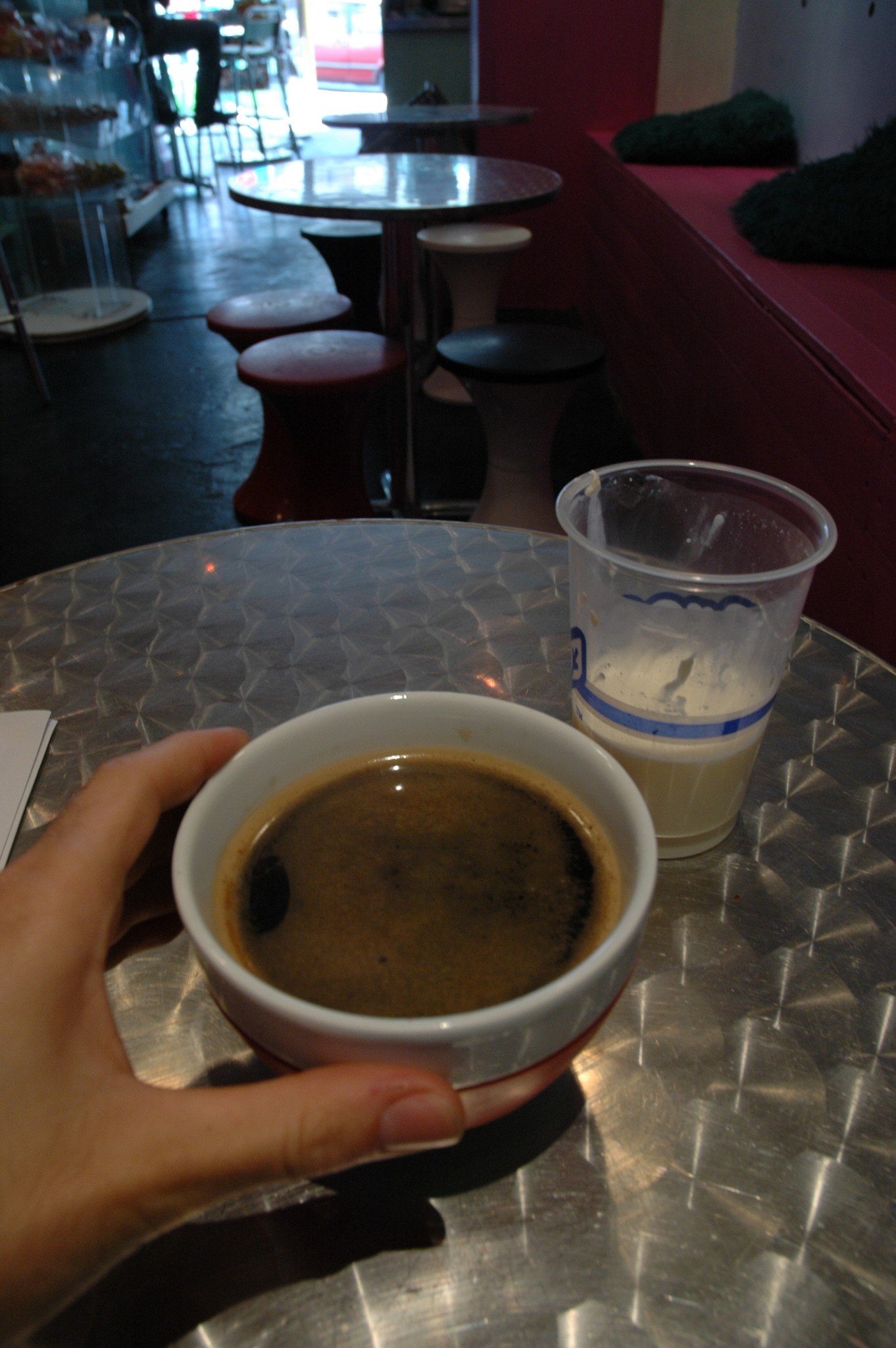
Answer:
[172,691,659,1131]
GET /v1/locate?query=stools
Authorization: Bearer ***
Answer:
[204,219,616,535]
[150,5,304,203]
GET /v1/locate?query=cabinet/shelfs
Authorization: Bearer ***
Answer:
[16,12,194,284]
[584,128,896,668]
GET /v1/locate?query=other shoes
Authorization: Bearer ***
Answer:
[152,104,180,124]
[194,109,238,128]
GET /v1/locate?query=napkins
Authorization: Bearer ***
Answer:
[1,710,56,875]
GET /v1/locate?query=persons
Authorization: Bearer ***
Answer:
[87,0,238,128]
[0,727,466,1348]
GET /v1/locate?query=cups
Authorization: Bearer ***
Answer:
[555,459,838,858]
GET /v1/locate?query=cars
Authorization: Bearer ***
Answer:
[312,36,386,95]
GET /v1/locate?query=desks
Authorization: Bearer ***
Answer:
[225,159,561,521]
[0,523,896,1347]
[327,110,535,358]
